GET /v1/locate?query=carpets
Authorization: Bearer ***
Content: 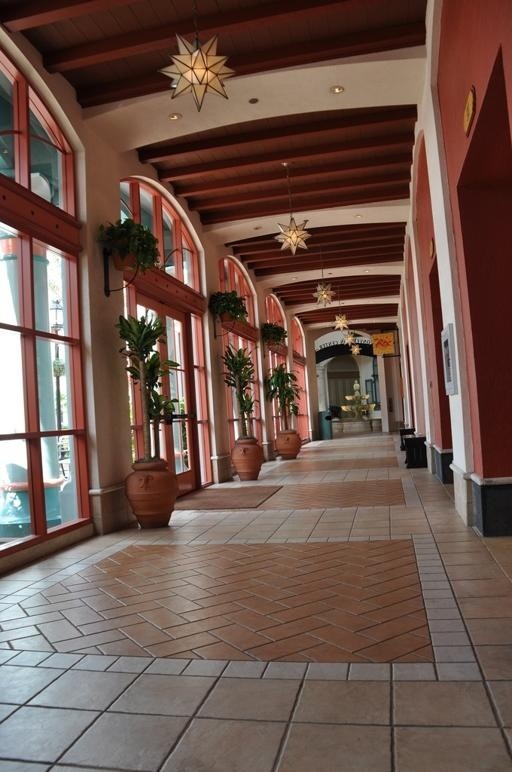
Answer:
[176,486,284,512]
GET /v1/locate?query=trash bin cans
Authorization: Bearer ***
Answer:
[318,412,332,439]
[400,428,426,469]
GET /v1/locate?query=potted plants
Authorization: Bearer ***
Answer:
[263,320,288,353]
[108,313,188,529]
[211,289,249,324]
[264,357,307,459]
[94,213,161,276]
[217,341,264,482]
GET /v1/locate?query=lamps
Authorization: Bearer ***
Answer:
[274,158,313,256]
[154,0,239,113]
[311,246,362,357]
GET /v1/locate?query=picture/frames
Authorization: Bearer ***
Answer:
[441,320,458,395]
